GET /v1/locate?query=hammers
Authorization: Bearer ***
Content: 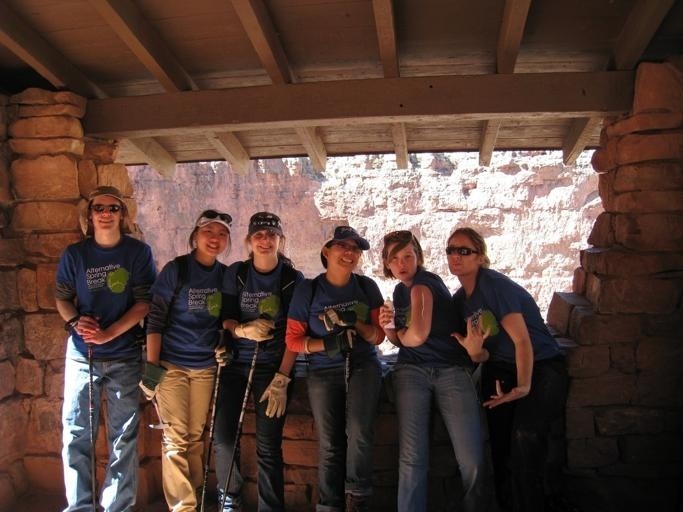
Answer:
[146,395,172,431]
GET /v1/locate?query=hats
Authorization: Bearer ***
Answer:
[320,226,371,269]
[196,209,232,234]
[248,212,283,238]
[89,186,125,202]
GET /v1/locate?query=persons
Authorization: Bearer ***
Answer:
[378,229,500,512]
[136,208,234,512]
[208,212,305,512]
[444,229,570,512]
[53,185,159,512]
[282,225,389,512]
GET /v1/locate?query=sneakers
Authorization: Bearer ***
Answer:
[217,484,244,512]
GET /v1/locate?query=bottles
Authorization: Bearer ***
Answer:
[383,296,396,329]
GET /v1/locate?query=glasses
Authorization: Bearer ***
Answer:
[446,246,480,256]
[90,203,122,212]
[251,216,281,228]
[196,211,232,227]
[383,230,413,246]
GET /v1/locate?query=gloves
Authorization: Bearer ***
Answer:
[139,362,167,400]
[235,318,275,342]
[318,309,357,332]
[259,373,292,419]
[214,343,234,368]
[324,329,357,359]
